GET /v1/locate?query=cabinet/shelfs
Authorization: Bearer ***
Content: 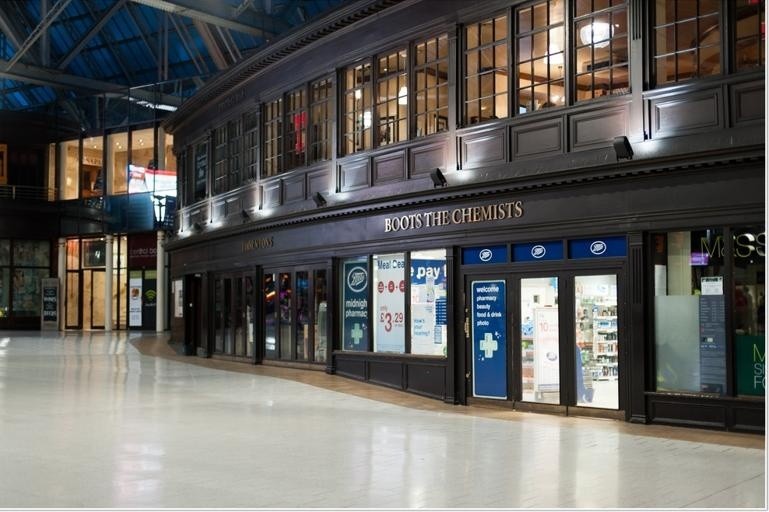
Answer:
[575,302,618,381]
[522,335,534,392]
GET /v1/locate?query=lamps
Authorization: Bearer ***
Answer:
[579,22,615,49]
[241,209,250,223]
[544,49,564,90]
[612,135,634,162]
[430,168,447,189]
[398,53,408,105]
[355,89,363,146]
[312,191,327,208]
